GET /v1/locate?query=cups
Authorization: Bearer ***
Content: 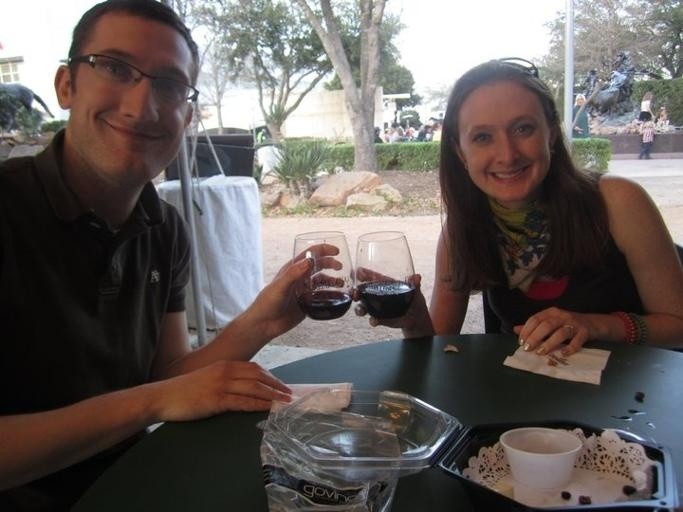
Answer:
[287,231,356,321]
[355,230,418,319]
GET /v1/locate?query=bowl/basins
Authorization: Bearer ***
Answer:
[498,427,584,487]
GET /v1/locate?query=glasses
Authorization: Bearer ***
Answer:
[71,51,200,109]
[499,56,539,80]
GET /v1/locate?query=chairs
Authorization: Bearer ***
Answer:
[482,238,683,356]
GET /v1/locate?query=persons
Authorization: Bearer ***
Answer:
[1,1,345,494]
[371,91,670,161]
[349,58,682,353]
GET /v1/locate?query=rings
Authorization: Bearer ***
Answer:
[563,322,576,338]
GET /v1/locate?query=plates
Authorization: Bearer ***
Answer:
[438,419,680,511]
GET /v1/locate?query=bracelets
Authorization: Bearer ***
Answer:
[611,311,649,345]
